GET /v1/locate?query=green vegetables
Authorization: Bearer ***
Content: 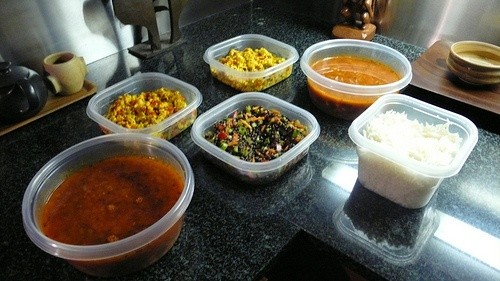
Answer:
[210,105,306,161]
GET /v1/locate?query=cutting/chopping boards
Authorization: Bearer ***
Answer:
[408,39,500,135]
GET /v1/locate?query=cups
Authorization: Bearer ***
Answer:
[43,52,88,96]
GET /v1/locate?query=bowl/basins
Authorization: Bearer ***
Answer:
[447,40,500,87]
[300,38,413,121]
[203,34,299,92]
[85,72,203,141]
[21,133,194,279]
[190,93,320,186]
[347,93,479,209]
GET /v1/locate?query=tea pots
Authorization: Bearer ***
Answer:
[0,60,48,122]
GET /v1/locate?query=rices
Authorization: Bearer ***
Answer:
[357,109,466,208]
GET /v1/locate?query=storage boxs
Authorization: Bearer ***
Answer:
[348,93,480,210]
